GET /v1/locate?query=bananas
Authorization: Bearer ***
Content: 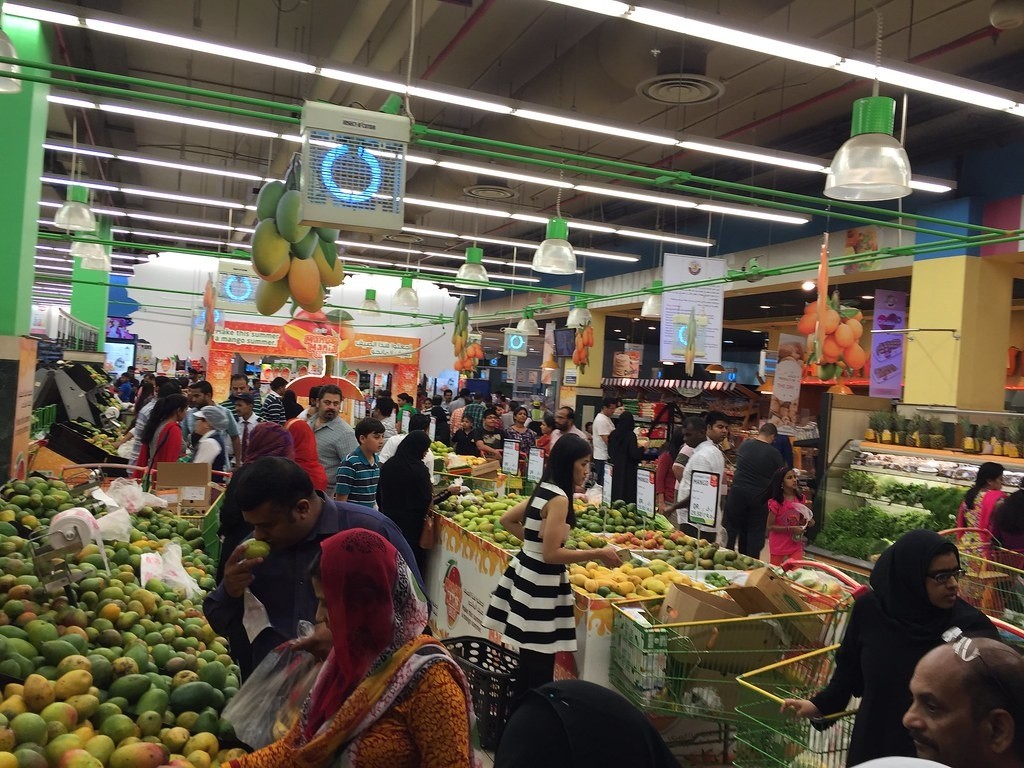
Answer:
[272,705,300,742]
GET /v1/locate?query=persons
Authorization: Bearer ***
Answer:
[657,410,728,544]
[902,637,1023,768]
[766,465,815,572]
[370,396,397,453]
[778,531,1003,768]
[332,416,387,507]
[373,431,461,561]
[988,477,1024,615]
[111,366,359,588]
[954,460,1007,618]
[203,455,433,680]
[592,398,615,485]
[482,432,622,689]
[221,528,477,768]
[378,413,435,488]
[389,385,592,476]
[719,421,782,560]
[605,412,646,509]
[369,388,398,421]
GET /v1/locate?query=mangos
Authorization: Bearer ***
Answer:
[796,300,865,382]
[433,484,847,599]
[429,441,453,456]
[282,309,345,349]
[572,326,593,366]
[0,476,272,768]
[252,181,345,314]
[71,392,127,456]
[455,343,484,371]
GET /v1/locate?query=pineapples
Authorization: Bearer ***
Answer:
[864,410,1024,458]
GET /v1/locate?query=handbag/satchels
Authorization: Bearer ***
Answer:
[105,477,168,513]
[92,508,130,542]
[141,540,204,605]
[418,483,435,549]
[221,620,324,750]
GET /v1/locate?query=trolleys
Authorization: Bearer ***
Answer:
[937,527,1024,653]
[732,610,1024,768]
[609,557,870,767]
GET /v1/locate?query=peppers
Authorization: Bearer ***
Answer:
[452,300,468,357]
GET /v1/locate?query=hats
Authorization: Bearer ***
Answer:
[232,393,254,405]
[192,406,229,431]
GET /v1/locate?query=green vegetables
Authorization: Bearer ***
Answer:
[814,470,971,563]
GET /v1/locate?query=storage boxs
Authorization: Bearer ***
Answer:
[25,362,138,490]
[155,462,213,516]
[609,566,825,717]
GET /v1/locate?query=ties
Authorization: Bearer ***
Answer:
[240,421,250,463]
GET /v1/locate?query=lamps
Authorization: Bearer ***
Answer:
[0,0,1024,329]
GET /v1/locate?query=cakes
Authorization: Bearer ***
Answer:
[615,354,630,376]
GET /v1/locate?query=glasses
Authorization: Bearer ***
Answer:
[194,418,201,420]
[941,625,1024,714]
[927,567,966,584]
